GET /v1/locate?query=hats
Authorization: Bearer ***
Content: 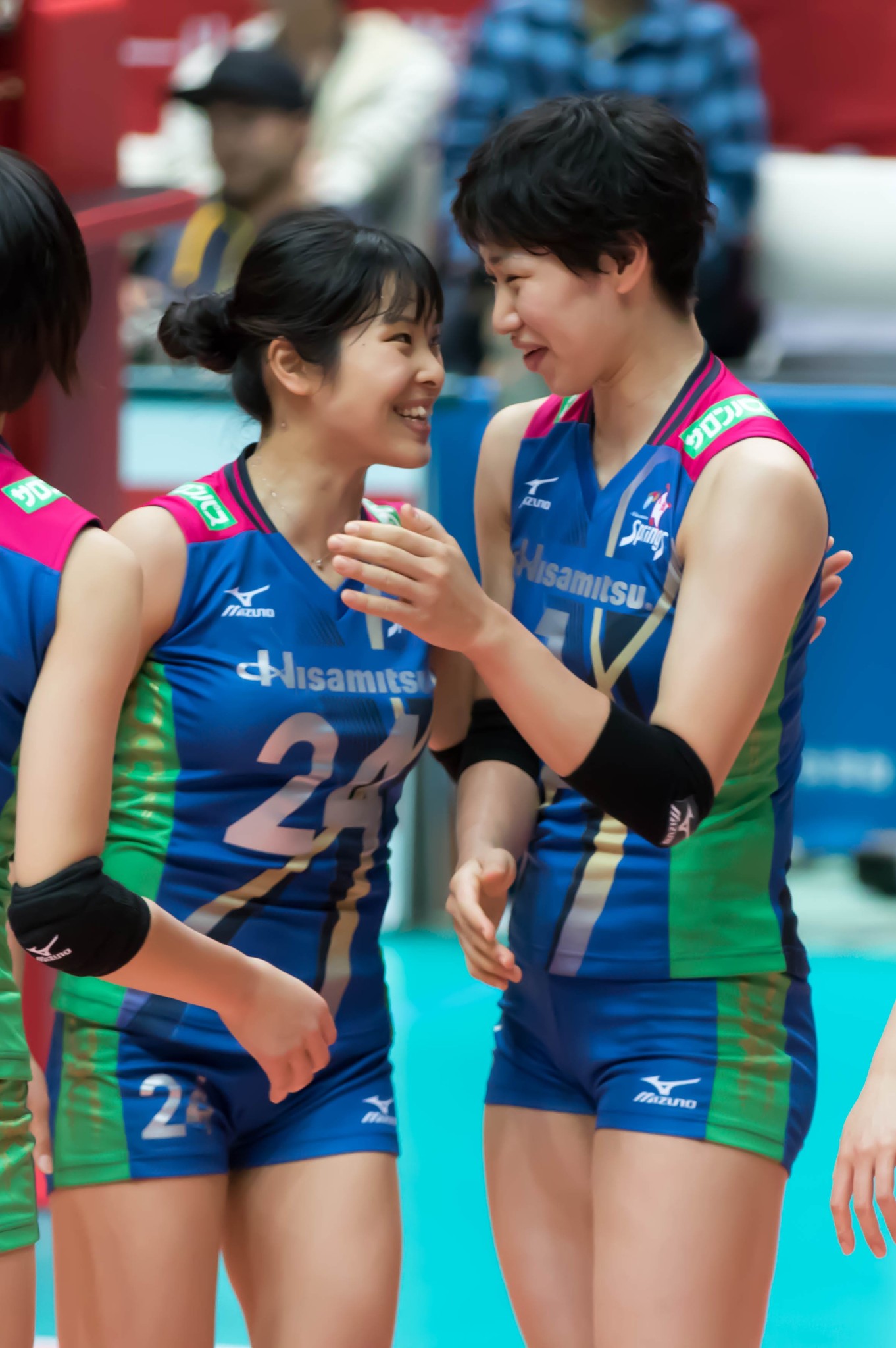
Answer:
[169,52,307,108]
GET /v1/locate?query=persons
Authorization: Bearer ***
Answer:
[325,96,828,1348]
[54,205,852,1348]
[832,996,895,1257]
[0,140,336,1346]
[119,0,766,368]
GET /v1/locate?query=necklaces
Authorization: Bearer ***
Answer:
[272,492,330,567]
